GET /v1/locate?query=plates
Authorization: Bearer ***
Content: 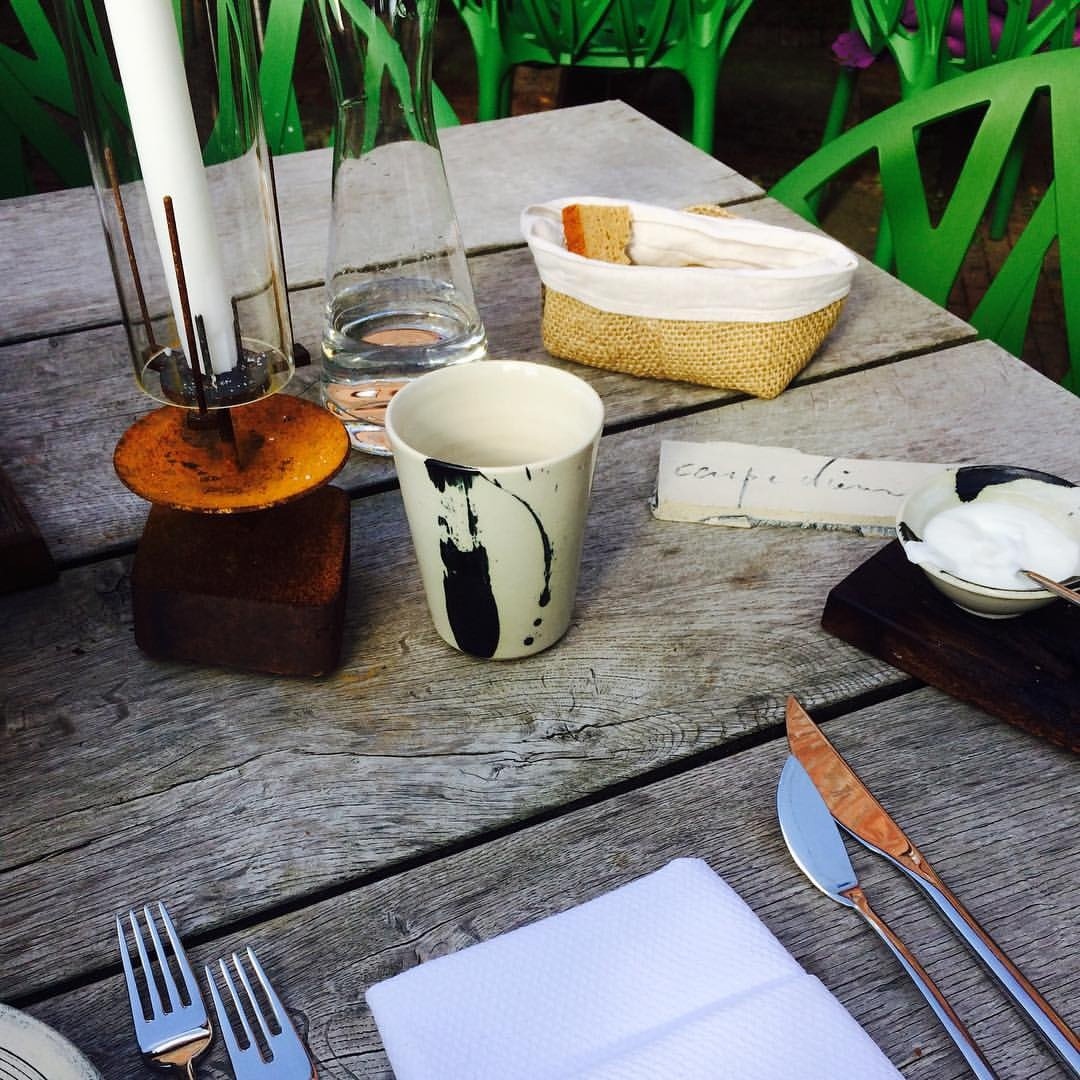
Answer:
[0,1005,102,1078]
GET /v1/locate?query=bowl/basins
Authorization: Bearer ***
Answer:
[897,463,1080,620]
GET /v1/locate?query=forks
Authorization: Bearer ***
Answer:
[205,946,316,1080]
[115,898,214,1080]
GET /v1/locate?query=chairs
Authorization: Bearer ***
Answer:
[0,0,1080,398]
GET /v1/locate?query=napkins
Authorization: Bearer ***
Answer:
[363,857,907,1080]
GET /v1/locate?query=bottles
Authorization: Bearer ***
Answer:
[311,0,490,460]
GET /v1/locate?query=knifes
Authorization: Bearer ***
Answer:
[775,756,1003,1080]
[783,693,1077,1079]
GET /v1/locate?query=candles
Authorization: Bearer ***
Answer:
[103,0,237,375]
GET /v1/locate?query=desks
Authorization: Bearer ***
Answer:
[0,100,1080,1080]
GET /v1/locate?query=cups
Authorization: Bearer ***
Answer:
[385,360,605,662]
